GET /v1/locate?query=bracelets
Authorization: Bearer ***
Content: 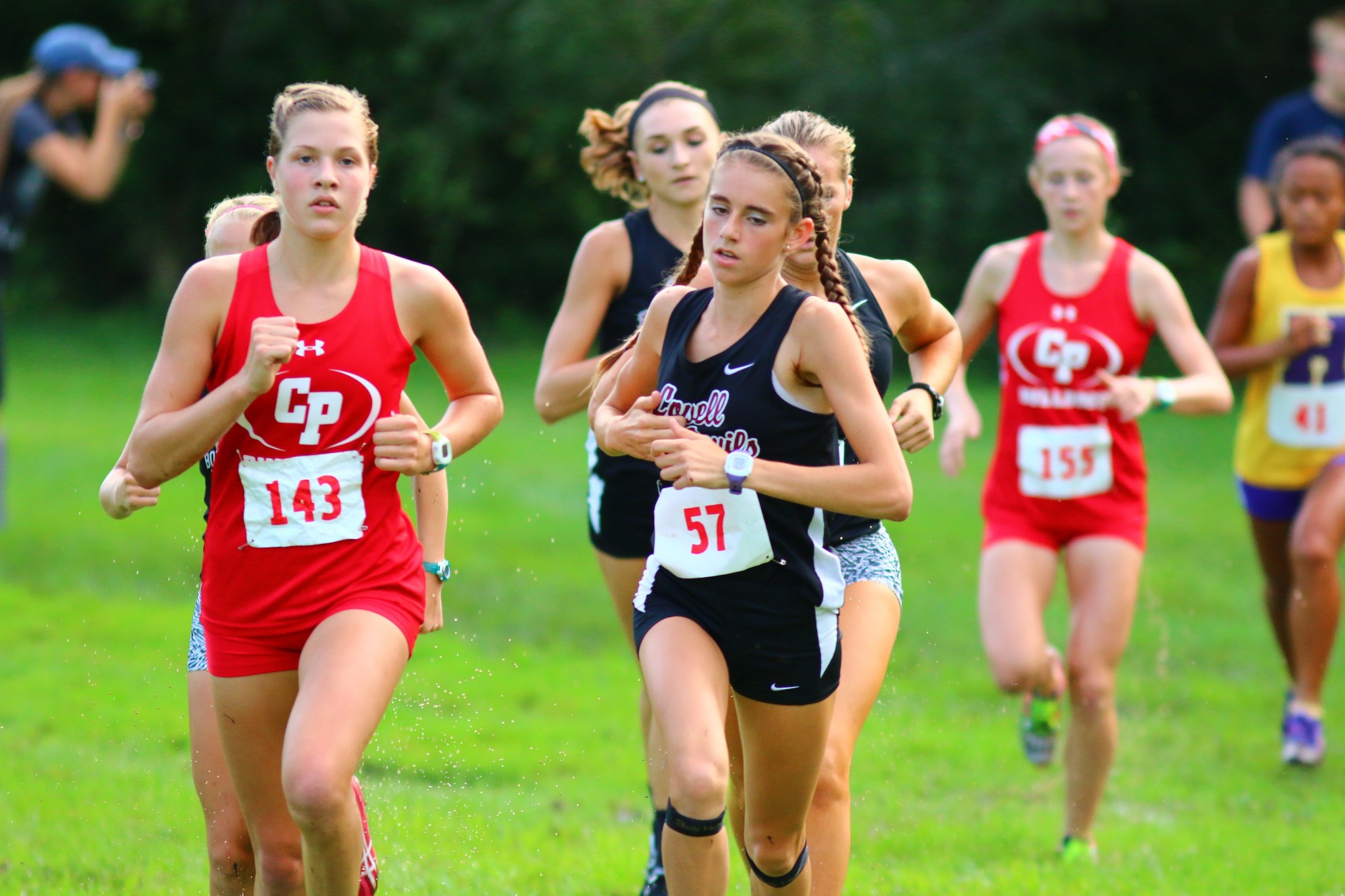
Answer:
[1155,372,1180,415]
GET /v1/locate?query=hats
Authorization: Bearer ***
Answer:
[35,25,139,81]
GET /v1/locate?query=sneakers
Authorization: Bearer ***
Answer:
[1058,834,1097,865]
[636,840,667,896]
[1023,646,1064,772]
[349,779,377,895]
[1279,698,1328,768]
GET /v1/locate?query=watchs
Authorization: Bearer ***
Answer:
[422,558,455,584]
[421,428,455,478]
[906,382,945,421]
[723,448,753,496]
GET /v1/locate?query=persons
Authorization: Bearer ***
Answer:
[532,76,964,896]
[1,21,159,297]
[98,79,508,896]
[941,114,1235,865]
[1235,11,1344,240]
[1204,138,1345,768]
